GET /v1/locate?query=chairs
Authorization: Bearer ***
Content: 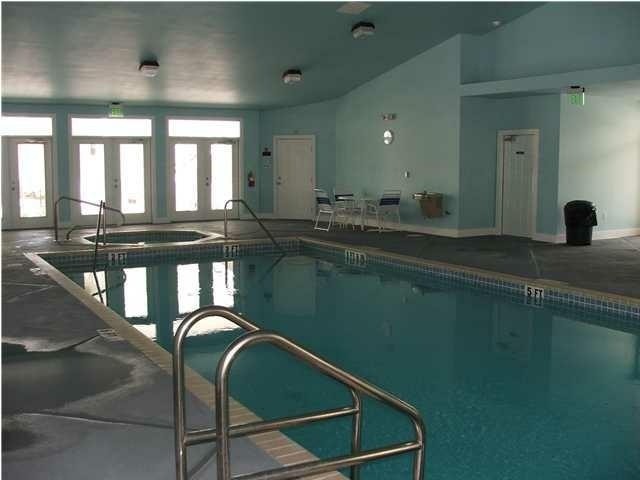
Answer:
[312,184,403,232]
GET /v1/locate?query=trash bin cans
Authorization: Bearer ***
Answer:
[564,200,597,246]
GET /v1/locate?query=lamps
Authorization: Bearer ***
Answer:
[351,21,375,40]
[282,70,302,84]
[139,61,159,78]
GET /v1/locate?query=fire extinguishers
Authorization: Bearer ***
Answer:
[248,172,255,187]
